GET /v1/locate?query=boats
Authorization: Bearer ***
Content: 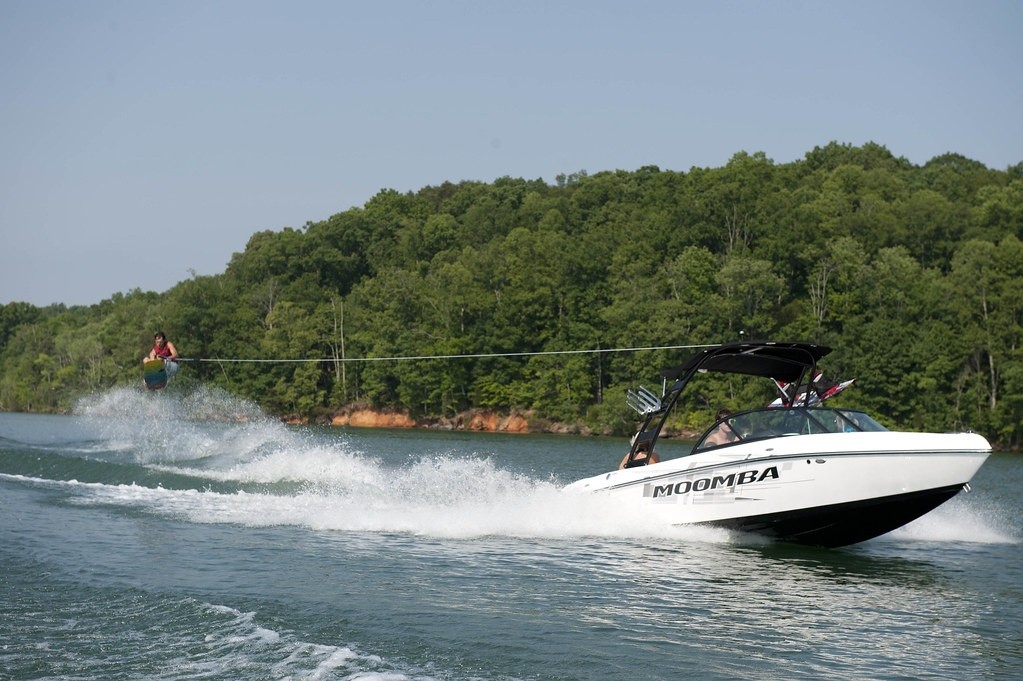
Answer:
[562,341,996,551]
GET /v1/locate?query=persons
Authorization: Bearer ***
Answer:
[619,431,661,471]
[142,331,180,389]
[702,407,745,449]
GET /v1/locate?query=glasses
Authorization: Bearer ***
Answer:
[728,419,736,425]
